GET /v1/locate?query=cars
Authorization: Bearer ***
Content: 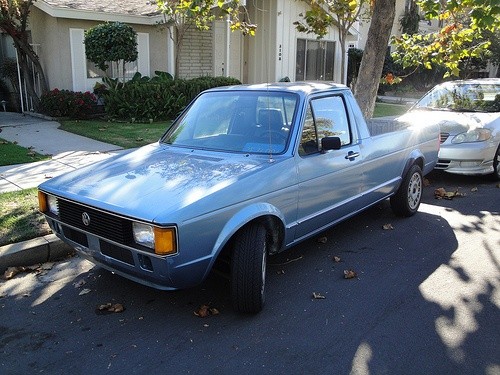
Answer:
[395,78,500,182]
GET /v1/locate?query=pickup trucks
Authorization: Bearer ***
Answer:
[37,80,440,309]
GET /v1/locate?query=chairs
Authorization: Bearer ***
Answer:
[255,109,285,138]
